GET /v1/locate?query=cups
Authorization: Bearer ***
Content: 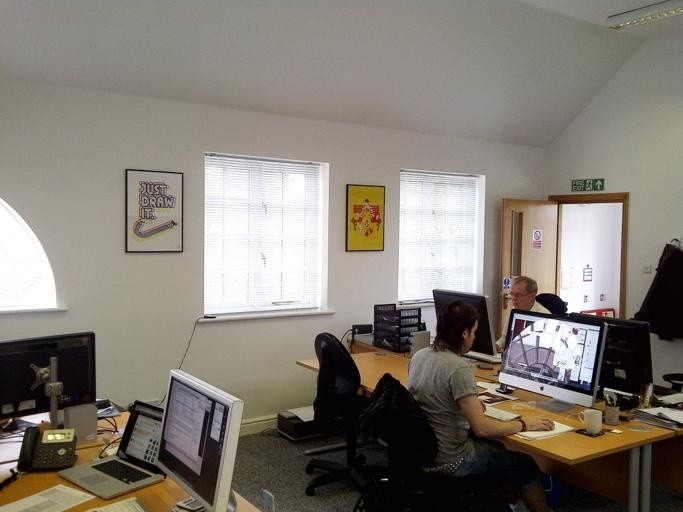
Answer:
[638,383,653,409]
[604,404,620,426]
[578,409,603,435]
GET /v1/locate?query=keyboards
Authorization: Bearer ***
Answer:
[463,350,502,363]
[484,406,521,422]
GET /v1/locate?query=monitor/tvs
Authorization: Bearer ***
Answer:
[569,313,653,400]
[0,332,96,420]
[433,290,497,356]
[155,370,245,512]
[499,309,608,408]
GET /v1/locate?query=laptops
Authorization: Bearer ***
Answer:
[58,401,167,499]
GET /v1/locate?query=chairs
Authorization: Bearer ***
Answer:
[305,333,369,496]
[533,294,568,320]
[353,377,510,511]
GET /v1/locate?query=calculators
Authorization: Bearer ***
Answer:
[176,497,203,511]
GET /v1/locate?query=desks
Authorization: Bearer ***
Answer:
[297,349,676,512]
[0,411,265,512]
[347,333,683,512]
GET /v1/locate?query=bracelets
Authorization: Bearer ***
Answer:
[519,420,526,432]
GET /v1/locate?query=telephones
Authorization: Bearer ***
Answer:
[17,427,77,472]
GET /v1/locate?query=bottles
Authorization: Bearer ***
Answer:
[420,320,426,331]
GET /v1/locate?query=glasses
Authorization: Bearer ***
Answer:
[507,290,538,298]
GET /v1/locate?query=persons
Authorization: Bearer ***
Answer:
[496,276,552,352]
[408,299,555,512]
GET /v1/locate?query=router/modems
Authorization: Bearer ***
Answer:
[410,331,430,355]
[64,404,97,445]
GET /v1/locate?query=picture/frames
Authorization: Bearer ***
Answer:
[345,184,385,252]
[583,267,592,281]
[124,168,184,254]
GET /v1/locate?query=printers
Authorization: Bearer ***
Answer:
[278,406,315,439]
